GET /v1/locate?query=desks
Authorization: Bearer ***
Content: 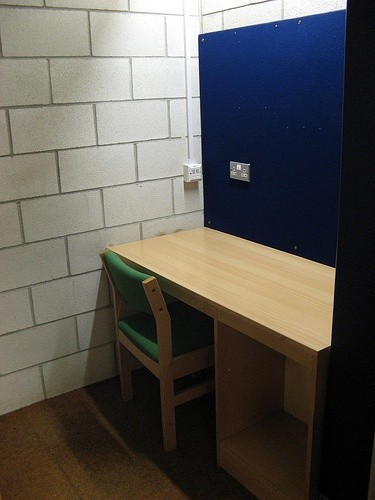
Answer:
[105,225,336,500]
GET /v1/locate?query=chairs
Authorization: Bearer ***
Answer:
[98,248,217,452]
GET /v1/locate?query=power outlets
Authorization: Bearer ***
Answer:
[183,163,201,183]
[230,161,251,182]
